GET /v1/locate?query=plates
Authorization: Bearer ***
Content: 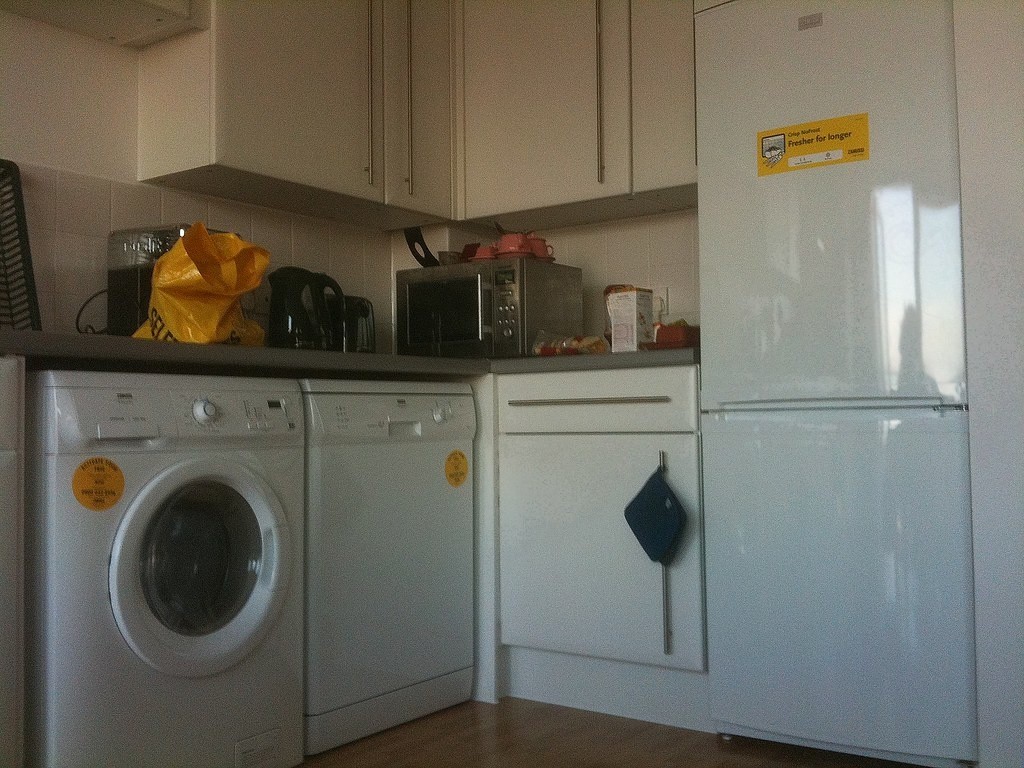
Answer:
[495,250,534,258]
[536,254,556,265]
[466,256,498,263]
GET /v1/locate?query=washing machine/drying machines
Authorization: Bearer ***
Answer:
[26,367,305,767]
[297,373,479,758]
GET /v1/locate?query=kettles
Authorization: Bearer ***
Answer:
[269,267,344,352]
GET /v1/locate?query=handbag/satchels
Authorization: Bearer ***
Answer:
[132,221,270,345]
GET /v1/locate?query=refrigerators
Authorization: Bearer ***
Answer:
[690,0,978,768]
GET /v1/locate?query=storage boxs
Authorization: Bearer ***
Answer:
[609,286,653,353]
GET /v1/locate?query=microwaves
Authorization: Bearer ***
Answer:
[397,260,583,361]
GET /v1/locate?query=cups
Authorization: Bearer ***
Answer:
[528,237,554,256]
[475,247,498,256]
[500,234,528,251]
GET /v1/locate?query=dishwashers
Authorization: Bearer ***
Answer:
[302,380,475,755]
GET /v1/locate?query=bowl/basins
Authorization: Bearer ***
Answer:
[438,251,459,264]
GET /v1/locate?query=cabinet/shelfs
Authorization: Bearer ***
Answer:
[496,365,712,734]
[0,0,209,48]
[135,0,452,232]
[450,0,700,234]
